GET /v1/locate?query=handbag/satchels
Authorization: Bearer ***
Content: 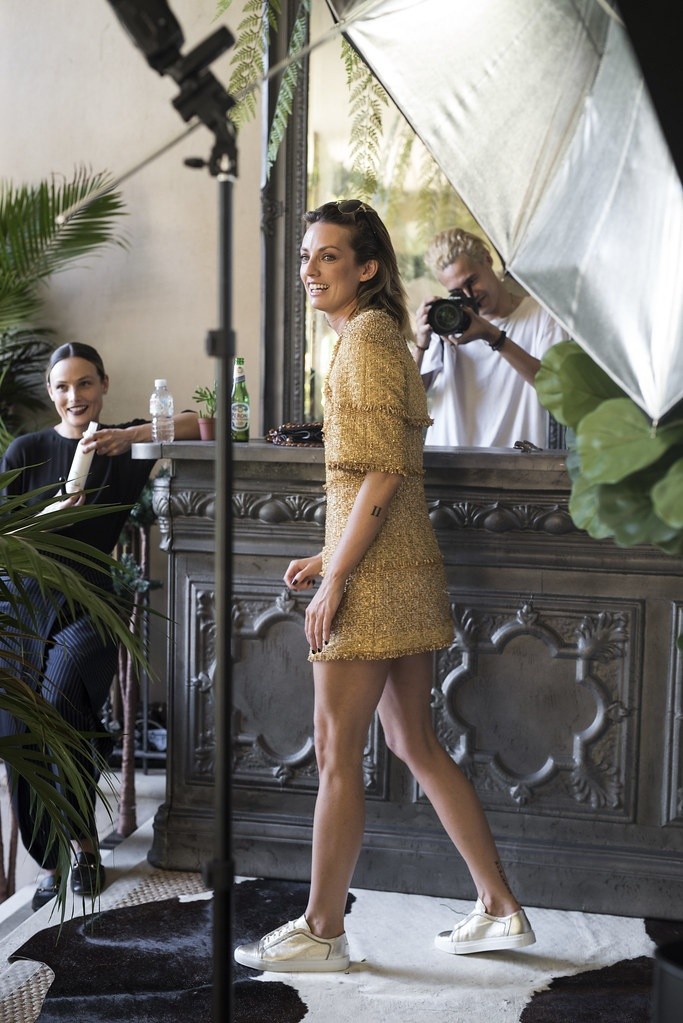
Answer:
[268,419,324,449]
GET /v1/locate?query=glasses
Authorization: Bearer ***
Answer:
[311,199,366,215]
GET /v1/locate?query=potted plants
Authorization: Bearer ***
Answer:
[191,383,217,442]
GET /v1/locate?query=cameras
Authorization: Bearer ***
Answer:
[426,288,479,337]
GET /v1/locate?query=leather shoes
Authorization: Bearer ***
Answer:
[32,873,64,911]
[70,851,105,894]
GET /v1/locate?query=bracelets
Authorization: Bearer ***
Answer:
[490,330,507,351]
[416,346,428,350]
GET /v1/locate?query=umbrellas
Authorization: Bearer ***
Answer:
[56,0,683,433]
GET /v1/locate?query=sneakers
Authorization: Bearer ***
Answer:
[233,915,349,971]
[434,897,535,954]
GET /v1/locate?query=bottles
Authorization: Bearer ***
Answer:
[228,357,251,441]
[66,421,99,496]
[149,378,176,444]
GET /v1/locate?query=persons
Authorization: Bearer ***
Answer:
[409,227,567,451]
[234,199,536,972]
[0,341,200,913]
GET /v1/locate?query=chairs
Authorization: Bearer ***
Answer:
[0,514,152,905]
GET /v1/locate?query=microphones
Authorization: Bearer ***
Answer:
[108,0,200,91]
